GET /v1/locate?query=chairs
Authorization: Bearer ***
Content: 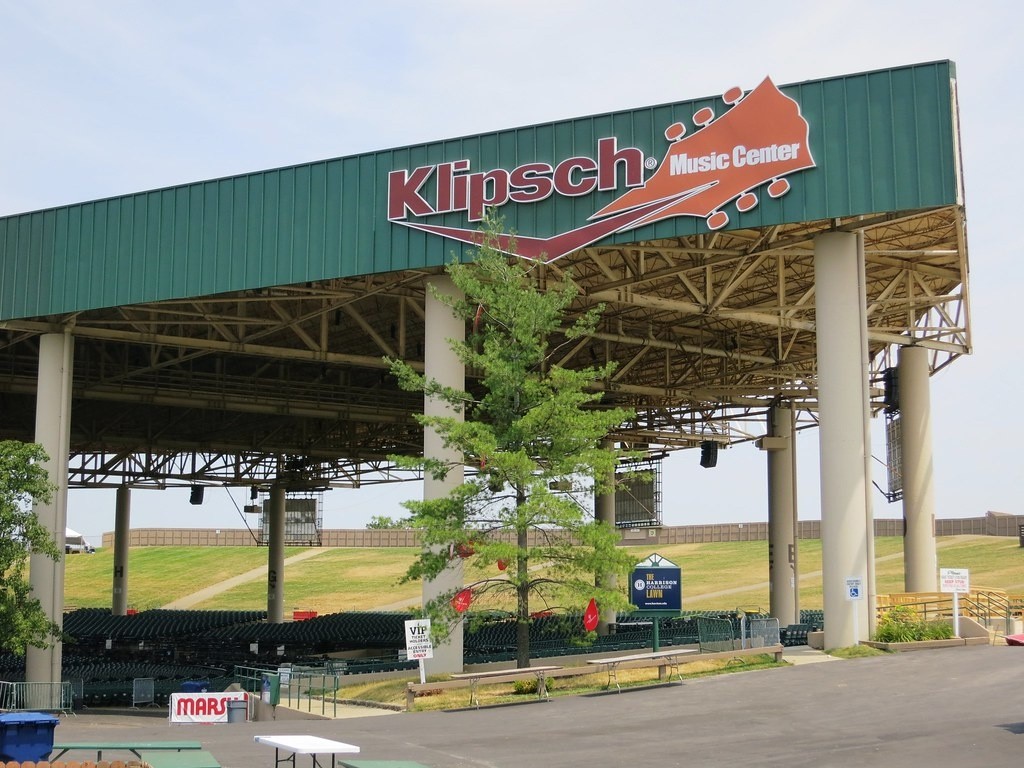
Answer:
[0,601,826,706]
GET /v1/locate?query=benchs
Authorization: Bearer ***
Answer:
[450,665,563,711]
[588,648,699,694]
[49,740,202,763]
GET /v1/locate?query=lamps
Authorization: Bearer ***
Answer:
[549,481,573,490]
[488,470,503,493]
[190,485,205,505]
[250,485,258,499]
[700,441,718,468]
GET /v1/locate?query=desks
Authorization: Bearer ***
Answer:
[141,749,221,768]
[608,621,653,635]
[336,758,430,768]
[254,733,361,768]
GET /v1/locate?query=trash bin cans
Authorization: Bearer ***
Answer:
[260,673,282,706]
[182,681,210,694]
[0,712,61,764]
[227,699,248,724]
